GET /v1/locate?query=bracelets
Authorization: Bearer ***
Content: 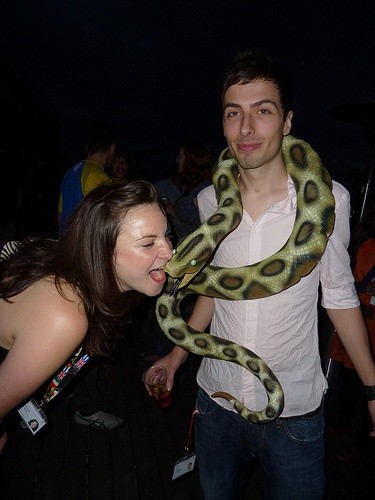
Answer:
[365,385,375,402]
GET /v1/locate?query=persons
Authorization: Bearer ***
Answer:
[0,177,173,450]
[59,125,123,429]
[108,146,132,186]
[143,54,375,500]
[162,140,210,373]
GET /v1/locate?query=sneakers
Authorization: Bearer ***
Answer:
[75,410,123,431]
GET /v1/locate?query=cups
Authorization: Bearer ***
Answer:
[142,365,172,408]
